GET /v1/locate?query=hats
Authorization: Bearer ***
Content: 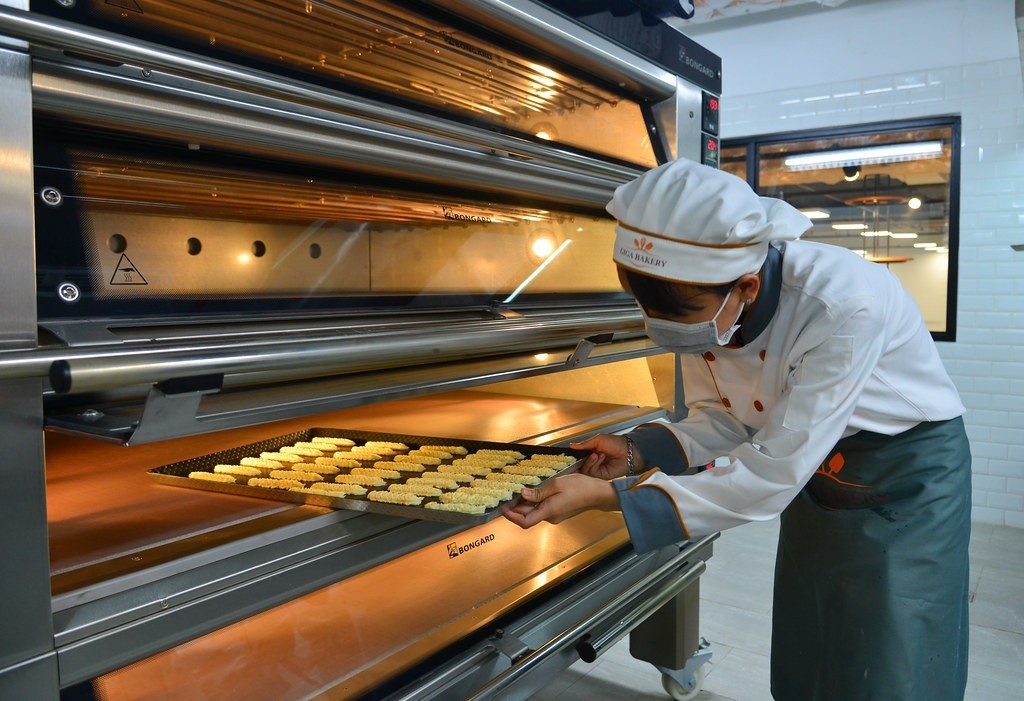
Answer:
[604,156,814,285]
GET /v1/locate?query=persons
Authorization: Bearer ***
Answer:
[503,157,972,700]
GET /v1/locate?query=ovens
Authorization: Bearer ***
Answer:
[5,0,727,701]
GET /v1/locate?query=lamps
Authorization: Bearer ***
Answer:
[785,138,948,254]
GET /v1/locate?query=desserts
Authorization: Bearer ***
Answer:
[188,436,578,515]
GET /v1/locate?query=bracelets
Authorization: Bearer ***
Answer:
[626,436,636,477]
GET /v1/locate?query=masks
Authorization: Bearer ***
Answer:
[634,285,747,354]
